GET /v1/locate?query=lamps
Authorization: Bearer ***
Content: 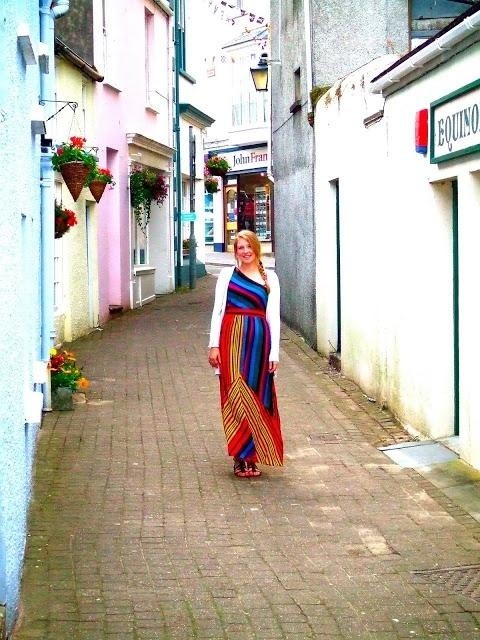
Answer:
[250,53,268,91]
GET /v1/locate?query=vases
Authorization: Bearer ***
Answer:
[52,387,75,410]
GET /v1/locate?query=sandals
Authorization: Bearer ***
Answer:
[233,462,261,478]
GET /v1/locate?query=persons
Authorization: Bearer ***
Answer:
[207,228,283,478]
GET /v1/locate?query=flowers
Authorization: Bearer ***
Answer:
[52,137,116,188]
[55,201,77,238]
[204,156,232,194]
[130,165,166,239]
[46,343,89,391]
